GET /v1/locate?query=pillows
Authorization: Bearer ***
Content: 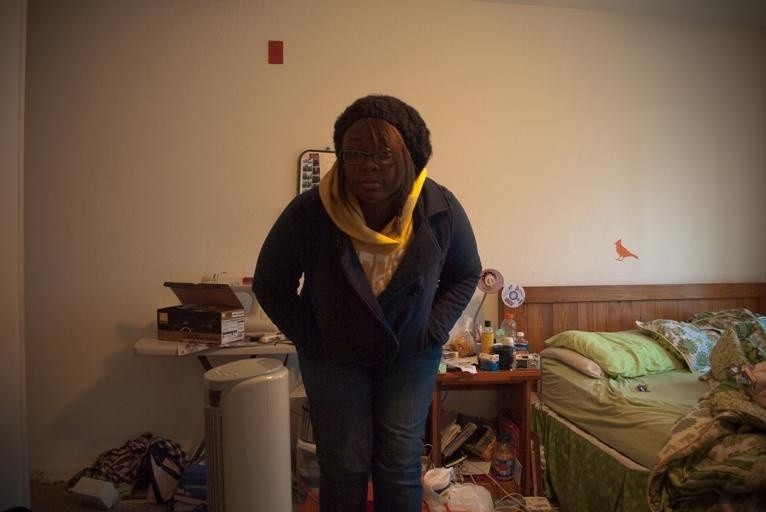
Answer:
[541,306,766,377]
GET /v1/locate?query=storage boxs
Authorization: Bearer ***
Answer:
[156,279,246,345]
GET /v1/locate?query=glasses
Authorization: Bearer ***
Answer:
[340,150,397,167]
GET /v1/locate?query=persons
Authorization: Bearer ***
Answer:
[251,96,481,512]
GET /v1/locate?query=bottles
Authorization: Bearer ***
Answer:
[481,311,530,371]
[494,432,516,483]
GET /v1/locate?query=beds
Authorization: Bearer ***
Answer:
[498,282,766,511]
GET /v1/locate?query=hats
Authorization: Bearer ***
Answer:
[333,95,432,177]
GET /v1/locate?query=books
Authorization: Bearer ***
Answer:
[173,484,210,512]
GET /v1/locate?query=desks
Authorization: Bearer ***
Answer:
[429,367,544,498]
[134,334,307,489]
